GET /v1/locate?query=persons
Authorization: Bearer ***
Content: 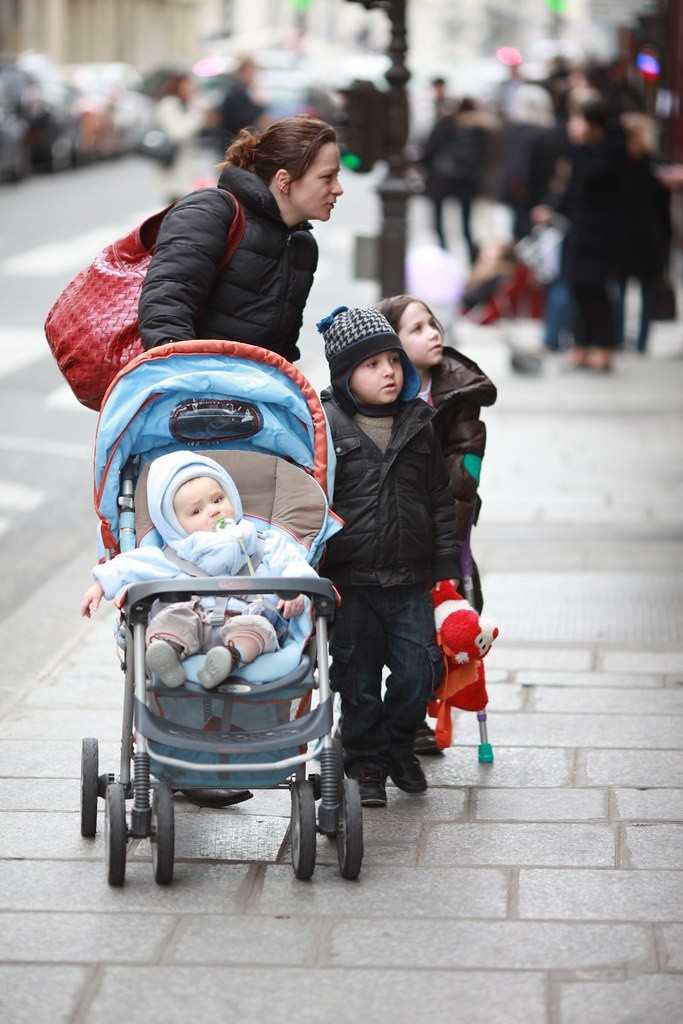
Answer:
[421,53,676,374]
[318,306,464,807]
[374,294,497,755]
[81,449,318,689]
[155,51,276,202]
[137,114,342,808]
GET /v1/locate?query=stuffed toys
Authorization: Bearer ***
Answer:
[430,579,499,711]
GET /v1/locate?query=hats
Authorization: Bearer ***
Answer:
[317,306,422,416]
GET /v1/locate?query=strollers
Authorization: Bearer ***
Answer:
[81,336,364,889]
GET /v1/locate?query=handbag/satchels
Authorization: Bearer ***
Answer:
[45,187,245,411]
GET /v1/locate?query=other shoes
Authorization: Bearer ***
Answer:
[387,758,427,793]
[414,724,443,753]
[565,345,610,371]
[360,769,387,804]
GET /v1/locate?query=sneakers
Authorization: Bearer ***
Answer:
[145,634,185,688]
[198,639,241,689]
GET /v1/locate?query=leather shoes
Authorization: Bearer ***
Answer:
[171,788,253,808]
